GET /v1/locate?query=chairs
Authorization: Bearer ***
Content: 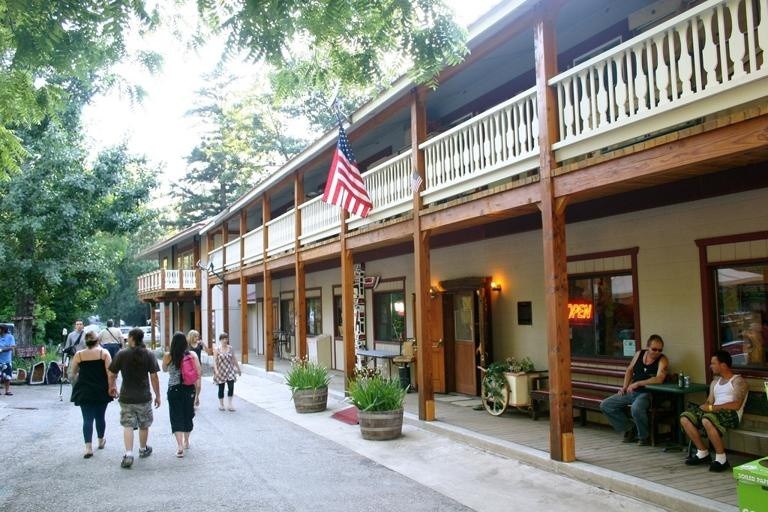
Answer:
[393,340,418,390]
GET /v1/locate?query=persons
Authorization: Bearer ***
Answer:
[680,349,749,473]
[70,330,117,459]
[598,334,668,446]
[0,324,15,395]
[63,320,88,387]
[212,332,242,412]
[82,316,102,337]
[99,320,125,359]
[163,331,201,459]
[108,328,160,467]
[743,319,764,363]
[187,329,214,407]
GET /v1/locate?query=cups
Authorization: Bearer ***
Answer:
[684,377,690,387]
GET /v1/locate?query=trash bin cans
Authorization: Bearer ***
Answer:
[733,457,768,512]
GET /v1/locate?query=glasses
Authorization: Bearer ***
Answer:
[649,346,664,352]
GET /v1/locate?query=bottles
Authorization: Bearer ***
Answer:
[678,368,684,389]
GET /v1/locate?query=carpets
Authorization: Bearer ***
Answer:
[329,405,360,426]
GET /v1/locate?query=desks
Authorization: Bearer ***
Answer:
[355,350,401,385]
[643,382,709,457]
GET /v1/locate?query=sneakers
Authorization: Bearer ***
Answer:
[120,455,134,467]
[708,458,729,473]
[139,444,153,458]
[622,428,650,446]
[685,453,713,466]
[6,391,14,395]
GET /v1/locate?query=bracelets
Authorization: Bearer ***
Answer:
[708,404,713,411]
[0,349,2,352]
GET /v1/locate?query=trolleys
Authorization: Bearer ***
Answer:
[474,362,543,417]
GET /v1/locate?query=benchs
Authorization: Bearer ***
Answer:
[687,385,767,459]
[530,364,646,428]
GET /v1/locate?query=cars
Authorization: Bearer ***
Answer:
[573,319,636,356]
[0,323,15,336]
[82,321,159,349]
[719,306,764,369]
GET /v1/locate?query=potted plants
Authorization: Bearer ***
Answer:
[285,355,332,414]
[344,375,412,442]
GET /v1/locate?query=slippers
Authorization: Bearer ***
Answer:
[174,448,185,457]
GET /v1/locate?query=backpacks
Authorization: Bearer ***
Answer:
[181,349,200,385]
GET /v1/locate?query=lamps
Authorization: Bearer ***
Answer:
[488,281,502,292]
[195,259,226,283]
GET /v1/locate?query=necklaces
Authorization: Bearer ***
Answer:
[644,351,655,371]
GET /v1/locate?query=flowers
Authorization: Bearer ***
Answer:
[481,356,536,407]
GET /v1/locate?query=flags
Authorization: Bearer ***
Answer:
[411,170,423,192]
[322,112,373,219]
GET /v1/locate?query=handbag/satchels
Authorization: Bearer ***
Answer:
[119,342,123,349]
[63,346,76,356]
